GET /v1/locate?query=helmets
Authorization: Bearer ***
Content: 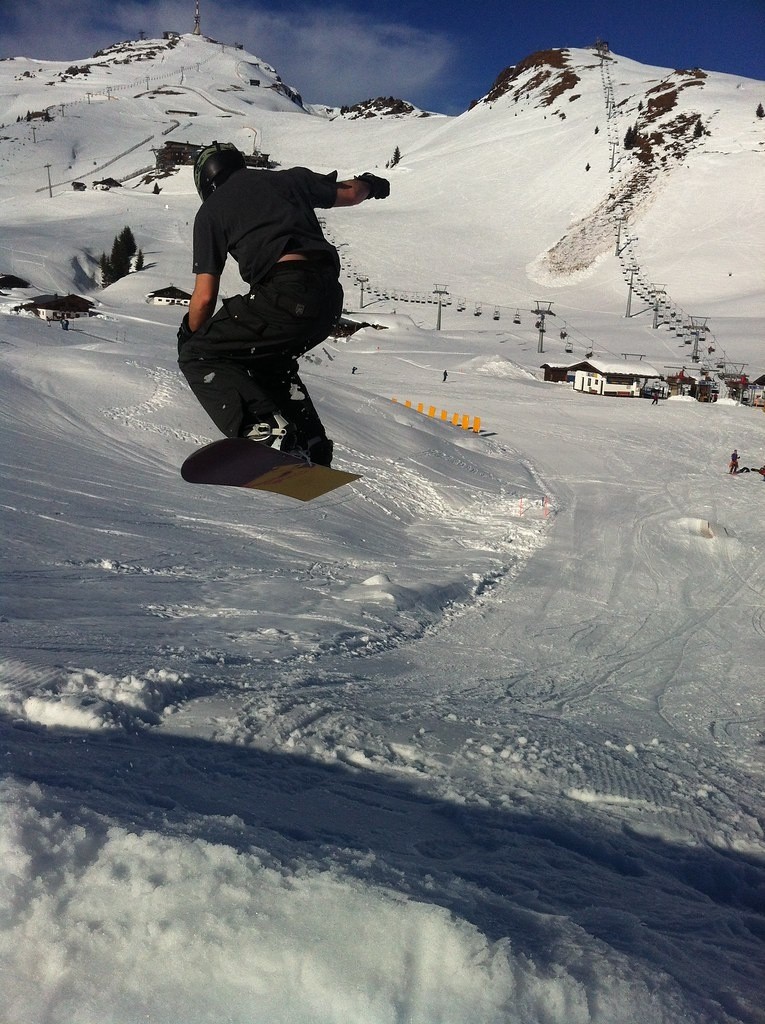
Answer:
[193,140,246,203]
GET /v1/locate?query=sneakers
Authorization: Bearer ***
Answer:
[295,429,334,468]
[239,413,296,453]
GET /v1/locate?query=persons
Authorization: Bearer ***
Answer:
[651,391,658,405]
[176,140,391,468]
[729,449,741,474]
[442,370,448,382]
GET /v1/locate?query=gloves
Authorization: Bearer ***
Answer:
[354,171,391,200]
[175,312,194,362]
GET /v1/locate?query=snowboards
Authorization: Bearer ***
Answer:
[180,437,364,503]
